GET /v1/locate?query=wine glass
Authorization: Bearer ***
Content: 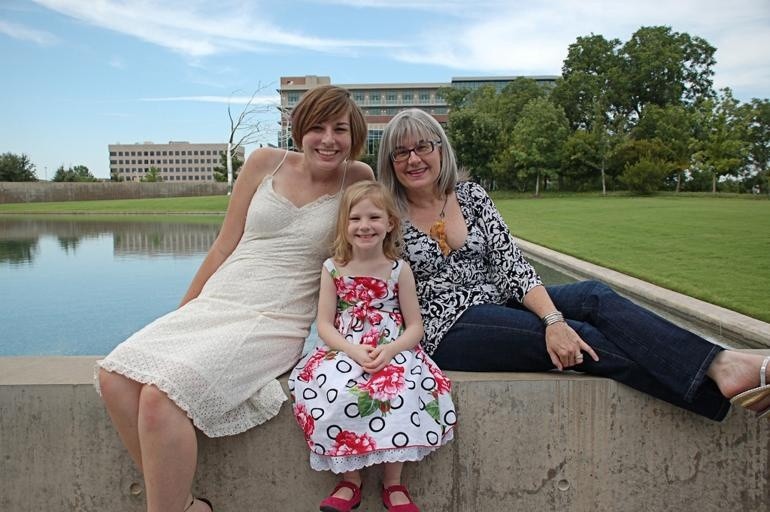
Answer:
[575,354,583,359]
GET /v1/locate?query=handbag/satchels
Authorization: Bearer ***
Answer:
[542,311,564,326]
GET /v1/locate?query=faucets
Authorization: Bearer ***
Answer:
[320,480,420,512]
[193,495,213,512]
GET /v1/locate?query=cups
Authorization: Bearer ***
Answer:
[391,140,441,161]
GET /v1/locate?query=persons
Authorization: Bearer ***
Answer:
[377,107,770,422]
[91,84,375,512]
[287,181,457,512]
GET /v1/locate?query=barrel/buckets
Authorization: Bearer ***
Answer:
[730,354,770,419]
